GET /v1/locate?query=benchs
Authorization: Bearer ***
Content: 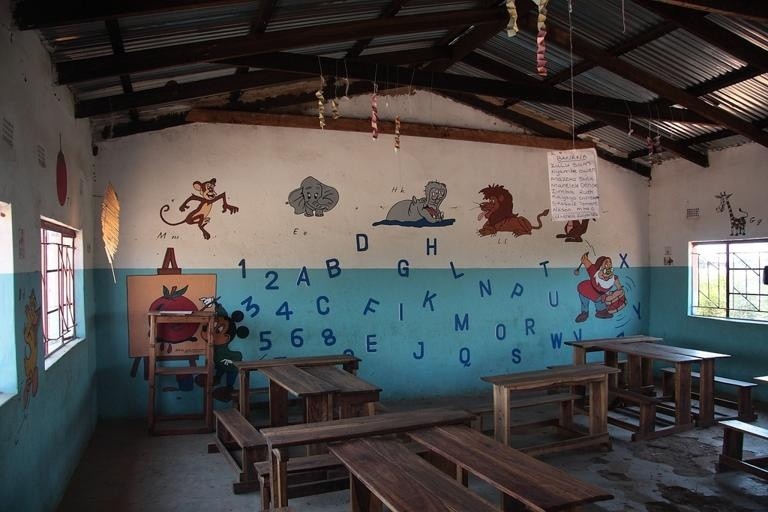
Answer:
[207,407,266,494]
[719,418,767,480]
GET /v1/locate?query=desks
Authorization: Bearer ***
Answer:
[260,405,616,511]
[567,334,758,443]
[236,352,383,454]
[480,364,622,453]
